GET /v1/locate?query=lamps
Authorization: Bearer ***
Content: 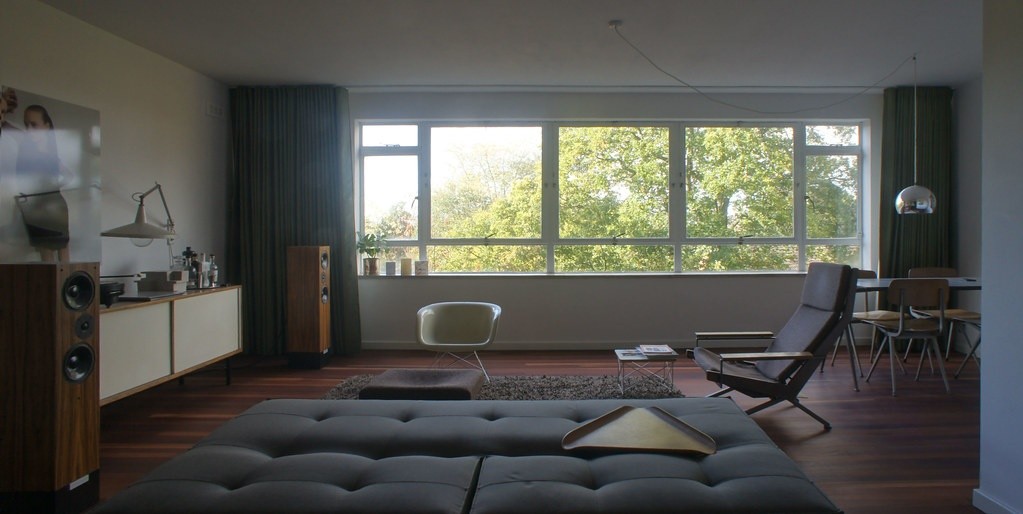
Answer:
[894,54,937,216]
[101,181,179,271]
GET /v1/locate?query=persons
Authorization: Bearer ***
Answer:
[16,105,70,263]
[0,84,31,263]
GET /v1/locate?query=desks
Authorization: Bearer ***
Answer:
[98,283,243,407]
[614,349,678,395]
[856,276,981,292]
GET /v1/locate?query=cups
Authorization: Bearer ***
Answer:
[401,259,411,275]
[386,262,395,276]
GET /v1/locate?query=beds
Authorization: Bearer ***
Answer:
[86,397,841,514]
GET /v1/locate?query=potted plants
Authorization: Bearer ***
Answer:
[355,230,391,276]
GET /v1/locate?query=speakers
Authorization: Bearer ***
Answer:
[0,260,101,514]
[285,245,332,365]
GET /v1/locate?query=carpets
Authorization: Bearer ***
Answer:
[322,373,685,400]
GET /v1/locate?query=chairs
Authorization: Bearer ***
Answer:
[950,311,981,378]
[414,301,502,383]
[686,262,858,433]
[821,268,906,379]
[902,267,980,363]
[862,278,951,396]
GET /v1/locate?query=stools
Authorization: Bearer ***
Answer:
[358,368,486,400]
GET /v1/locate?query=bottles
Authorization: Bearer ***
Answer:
[173,246,219,289]
